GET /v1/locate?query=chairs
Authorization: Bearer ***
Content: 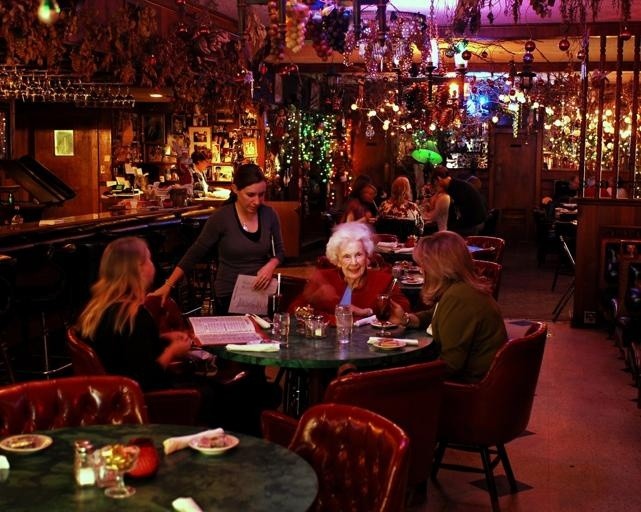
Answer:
[143,292,189,335]
[484,208,501,236]
[432,320,547,511]
[325,359,448,508]
[467,235,506,262]
[472,247,495,261]
[373,219,421,242]
[375,233,400,244]
[286,402,410,512]
[65,327,201,425]
[0,377,152,438]
[471,259,503,301]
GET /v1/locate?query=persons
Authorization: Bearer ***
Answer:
[288,222,410,327]
[380,177,424,234]
[79,236,284,427]
[542,174,627,204]
[145,164,284,316]
[185,148,213,194]
[383,231,509,385]
[433,166,488,235]
[425,176,450,232]
[339,184,377,224]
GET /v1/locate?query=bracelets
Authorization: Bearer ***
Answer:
[165,279,177,288]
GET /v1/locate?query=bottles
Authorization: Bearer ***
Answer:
[71,439,96,487]
[305,315,314,339]
[314,315,326,339]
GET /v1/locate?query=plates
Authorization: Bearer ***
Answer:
[189,433,238,456]
[401,279,423,285]
[371,319,410,328]
[374,341,406,351]
[0,434,53,455]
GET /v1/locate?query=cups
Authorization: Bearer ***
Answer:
[336,314,353,344]
[336,304,352,314]
[375,295,392,336]
[273,312,290,345]
[101,444,140,499]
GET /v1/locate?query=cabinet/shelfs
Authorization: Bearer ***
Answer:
[570,196,640,327]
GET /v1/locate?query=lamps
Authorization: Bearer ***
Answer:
[37,1,60,27]
[449,79,460,100]
[454,43,468,71]
[426,40,438,68]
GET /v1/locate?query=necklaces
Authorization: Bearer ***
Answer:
[243,214,252,231]
